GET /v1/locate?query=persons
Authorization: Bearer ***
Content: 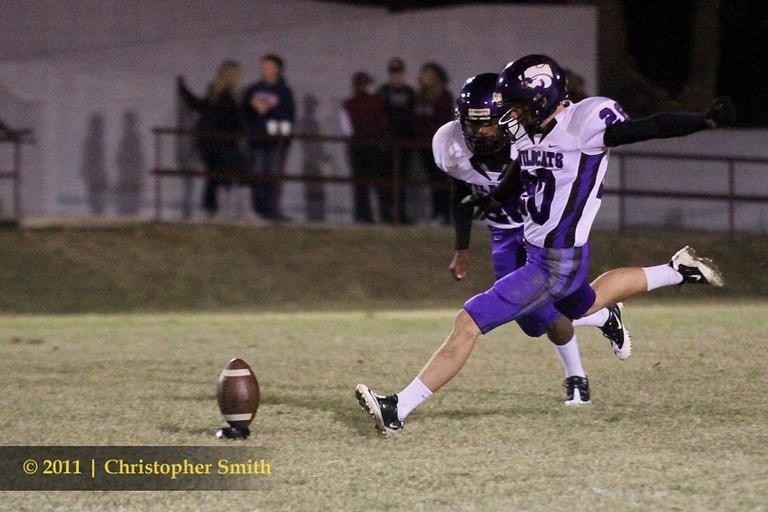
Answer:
[412,63,456,226]
[238,54,298,220]
[355,53,741,434]
[375,56,423,226]
[192,58,250,219]
[429,72,635,404]
[341,71,396,227]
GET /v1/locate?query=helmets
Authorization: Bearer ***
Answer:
[456,73,509,155]
[496,54,569,145]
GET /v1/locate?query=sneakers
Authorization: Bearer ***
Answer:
[355,384,404,440]
[597,301,630,361]
[668,246,724,289]
[565,375,590,407]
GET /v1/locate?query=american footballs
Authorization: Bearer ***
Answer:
[217,358,259,429]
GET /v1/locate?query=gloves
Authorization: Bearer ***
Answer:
[702,97,735,128]
[459,193,501,217]
